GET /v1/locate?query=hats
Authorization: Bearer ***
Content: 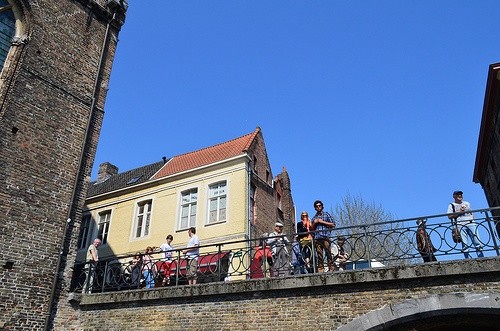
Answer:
[453,190,463,198]
[416,219,427,228]
[275,223,283,227]
[336,237,346,244]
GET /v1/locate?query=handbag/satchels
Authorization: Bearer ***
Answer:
[300,236,311,247]
[452,228,462,243]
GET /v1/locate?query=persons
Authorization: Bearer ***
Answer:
[416,218,437,263]
[132,252,142,288]
[82,238,102,294]
[159,234,175,287]
[311,200,336,273]
[331,236,349,272]
[142,247,155,289]
[447,190,485,259]
[181,227,200,285]
[259,211,315,278]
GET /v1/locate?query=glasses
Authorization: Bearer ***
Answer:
[315,205,322,208]
[302,215,308,217]
